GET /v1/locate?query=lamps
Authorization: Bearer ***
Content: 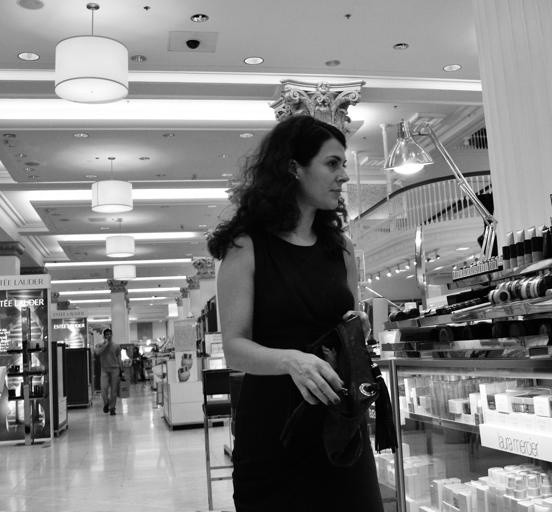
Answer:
[91,157,133,215]
[382,119,500,269]
[366,248,442,284]
[106,218,135,258]
[54,2,129,105]
[113,264,136,282]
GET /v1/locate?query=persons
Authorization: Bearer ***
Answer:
[132,346,145,384]
[94,328,124,415]
[206,115,384,511]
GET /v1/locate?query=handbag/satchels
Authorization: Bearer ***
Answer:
[313,315,400,471]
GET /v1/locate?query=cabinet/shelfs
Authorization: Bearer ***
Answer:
[150,299,551,511]
[7,308,47,446]
[34,429,35,432]
